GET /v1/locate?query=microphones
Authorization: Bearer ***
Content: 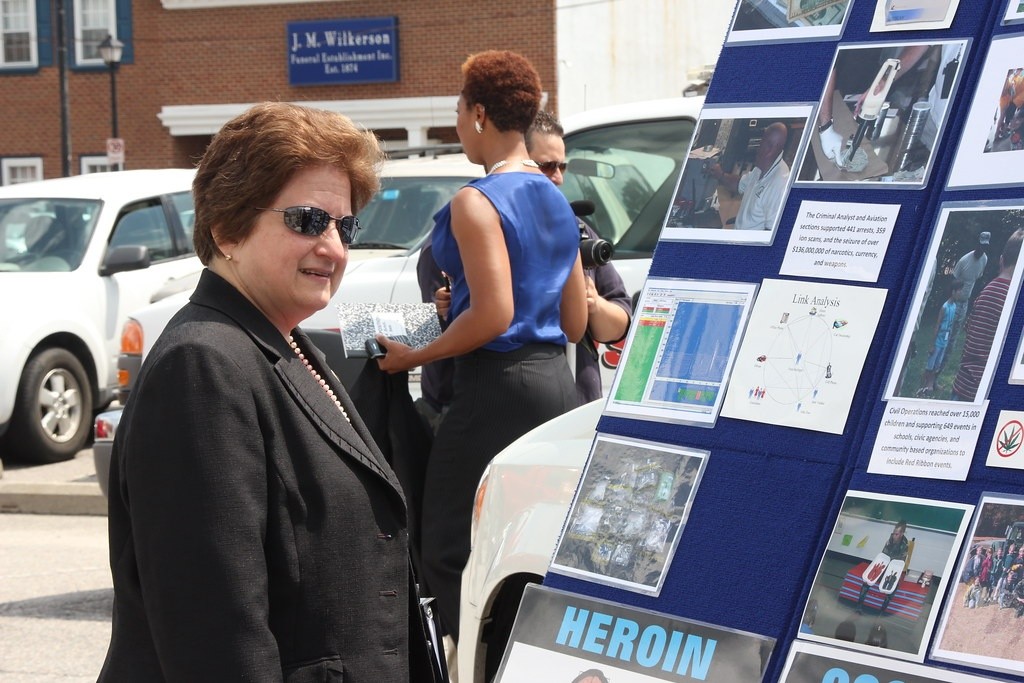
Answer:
[568,199,595,217]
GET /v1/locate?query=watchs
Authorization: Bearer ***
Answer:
[818,118,834,132]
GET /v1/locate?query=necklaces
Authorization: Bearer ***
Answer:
[486,157,537,174]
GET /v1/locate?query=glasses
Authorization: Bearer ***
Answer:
[531,160,568,175]
[254,205,362,245]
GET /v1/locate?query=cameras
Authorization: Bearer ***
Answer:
[579,223,613,270]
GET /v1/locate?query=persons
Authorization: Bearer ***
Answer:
[416,108,632,402]
[374,48,596,669]
[855,43,965,157]
[701,122,792,231]
[954,227,1024,400]
[852,521,908,622]
[96,103,411,683]
[949,230,994,369]
[965,541,1024,618]
[817,68,845,166]
[916,277,960,394]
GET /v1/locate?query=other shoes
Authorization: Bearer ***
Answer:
[999,131,1011,139]
[1000,125,1015,132]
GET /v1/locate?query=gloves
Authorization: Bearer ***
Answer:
[818,117,843,167]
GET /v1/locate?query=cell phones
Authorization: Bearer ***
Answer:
[365,335,413,359]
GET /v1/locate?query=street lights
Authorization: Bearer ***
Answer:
[96,32,125,170]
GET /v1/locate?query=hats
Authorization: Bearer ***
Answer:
[980,231,992,245]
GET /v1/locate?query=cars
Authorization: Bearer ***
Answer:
[297,94,710,401]
[457,392,609,683]
[0,168,207,462]
